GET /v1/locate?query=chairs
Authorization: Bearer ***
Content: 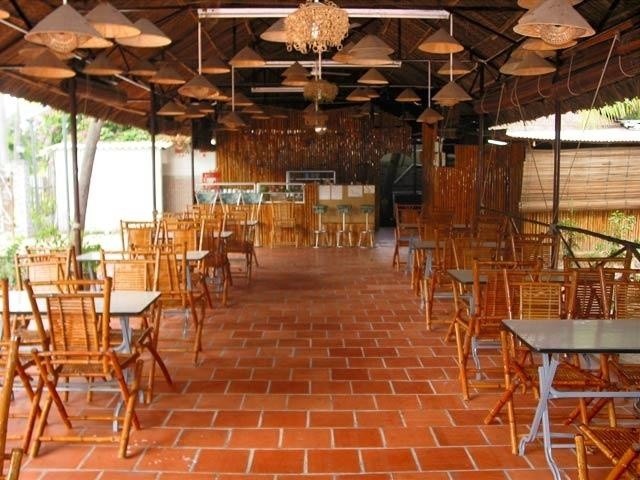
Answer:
[270,204,299,249]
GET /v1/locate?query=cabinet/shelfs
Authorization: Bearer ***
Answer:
[257,182,304,204]
[195,182,256,204]
[285,170,336,191]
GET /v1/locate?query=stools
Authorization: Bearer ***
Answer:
[359,204,376,248]
[335,203,355,252]
[310,204,329,248]
